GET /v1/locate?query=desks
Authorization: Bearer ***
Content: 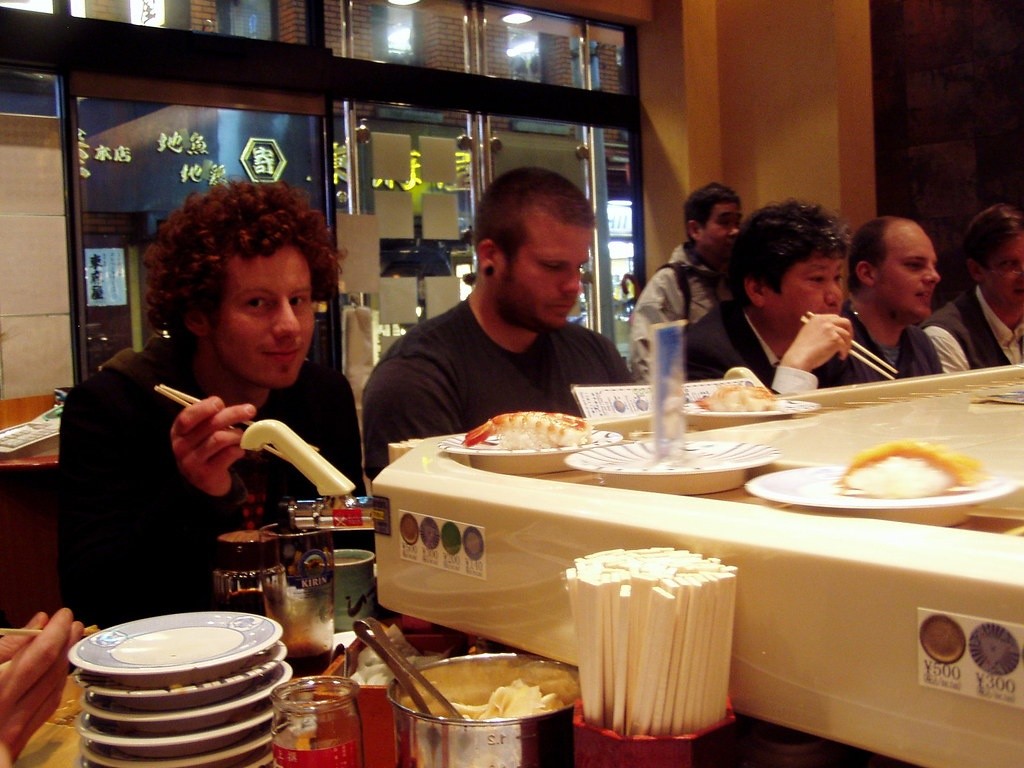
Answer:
[0,395,60,629]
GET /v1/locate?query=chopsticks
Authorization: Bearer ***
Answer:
[801,311,899,380]
[154,384,320,463]
[564,546,738,735]
[0,625,101,671]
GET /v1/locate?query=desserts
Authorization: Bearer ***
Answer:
[837,443,979,496]
[697,387,775,412]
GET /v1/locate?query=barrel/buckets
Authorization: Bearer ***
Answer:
[388,651,579,768]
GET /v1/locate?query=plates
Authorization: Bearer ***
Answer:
[68,611,292,768]
[746,465,1024,526]
[565,440,783,495]
[439,431,623,475]
[687,400,821,429]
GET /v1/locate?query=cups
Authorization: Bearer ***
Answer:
[260,523,334,677]
[269,675,363,768]
[572,698,736,768]
[333,549,375,631]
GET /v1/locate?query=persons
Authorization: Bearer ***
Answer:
[56,183,371,633]
[920,203,1024,373]
[843,216,943,383]
[685,202,854,394]
[631,182,742,382]
[361,166,633,481]
